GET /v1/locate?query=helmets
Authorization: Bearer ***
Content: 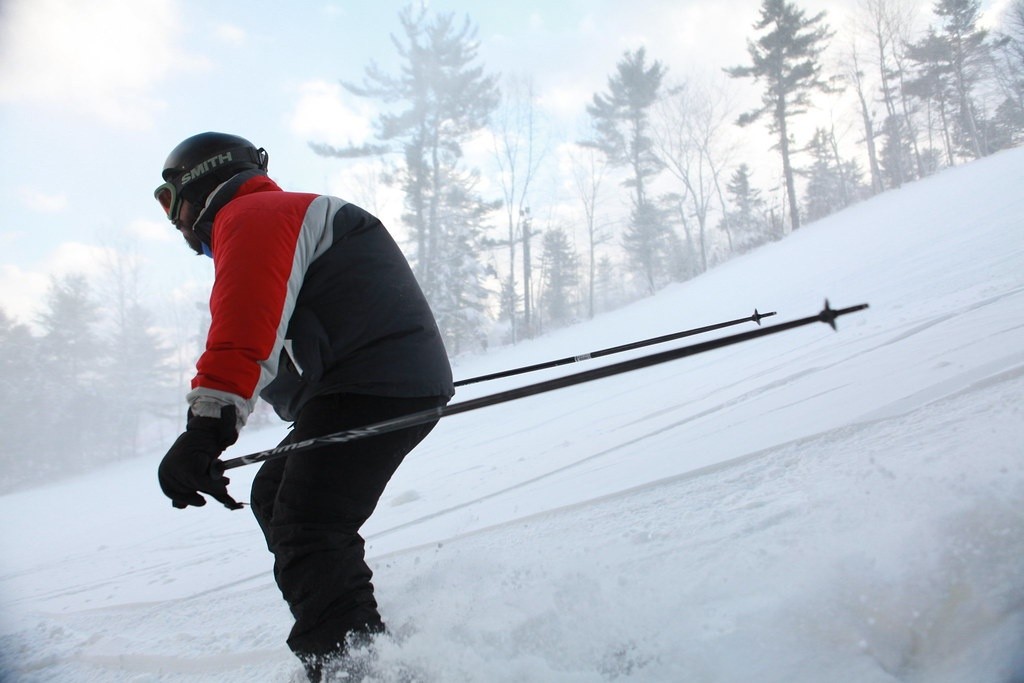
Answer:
[154,131,269,230]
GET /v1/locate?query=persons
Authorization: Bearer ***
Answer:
[156,131,457,683]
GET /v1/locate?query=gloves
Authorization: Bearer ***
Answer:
[158,403,239,509]
